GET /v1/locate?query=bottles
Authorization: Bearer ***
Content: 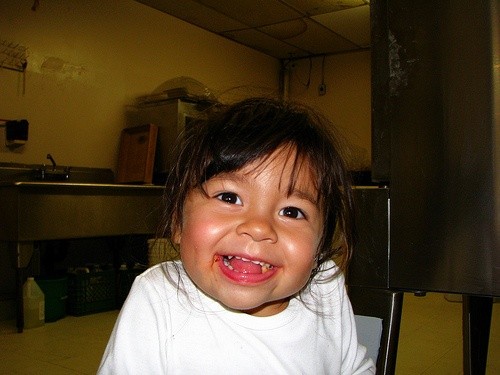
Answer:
[21,277,46,329]
[67,262,145,274]
[17,120,29,141]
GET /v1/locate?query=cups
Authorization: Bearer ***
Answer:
[5,119,18,140]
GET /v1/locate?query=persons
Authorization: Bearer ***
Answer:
[95,97,376,375]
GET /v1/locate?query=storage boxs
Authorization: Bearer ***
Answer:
[66,266,120,317]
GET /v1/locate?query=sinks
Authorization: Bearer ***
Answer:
[0,177,166,243]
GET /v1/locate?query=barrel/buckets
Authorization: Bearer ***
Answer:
[36,277,68,322]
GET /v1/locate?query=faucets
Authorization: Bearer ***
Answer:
[41,163,47,180]
